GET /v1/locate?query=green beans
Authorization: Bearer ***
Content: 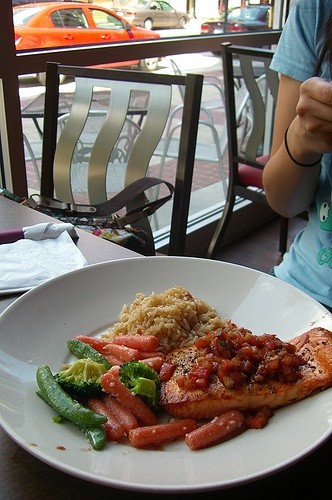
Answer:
[35,338,114,450]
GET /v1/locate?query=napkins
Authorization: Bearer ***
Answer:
[0,230,88,295]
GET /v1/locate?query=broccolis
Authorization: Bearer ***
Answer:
[117,359,163,412]
[50,357,110,403]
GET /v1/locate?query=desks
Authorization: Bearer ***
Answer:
[233,66,265,153]
[21,91,150,164]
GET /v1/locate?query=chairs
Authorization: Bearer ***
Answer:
[251,10,259,17]
[64,13,82,25]
[208,42,288,259]
[35,62,204,256]
[0,59,270,222]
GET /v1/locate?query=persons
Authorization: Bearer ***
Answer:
[262,0,332,310]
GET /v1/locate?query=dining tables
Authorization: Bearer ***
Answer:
[0,195,147,314]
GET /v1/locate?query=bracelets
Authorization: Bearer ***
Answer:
[284,127,324,167]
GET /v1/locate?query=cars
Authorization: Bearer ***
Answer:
[107,0,190,30]
[200,4,273,55]
[13,2,160,86]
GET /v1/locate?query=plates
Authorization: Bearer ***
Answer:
[0,255,332,492]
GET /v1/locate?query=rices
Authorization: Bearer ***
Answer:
[97,286,238,353]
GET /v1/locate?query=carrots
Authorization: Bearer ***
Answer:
[73,335,244,451]
